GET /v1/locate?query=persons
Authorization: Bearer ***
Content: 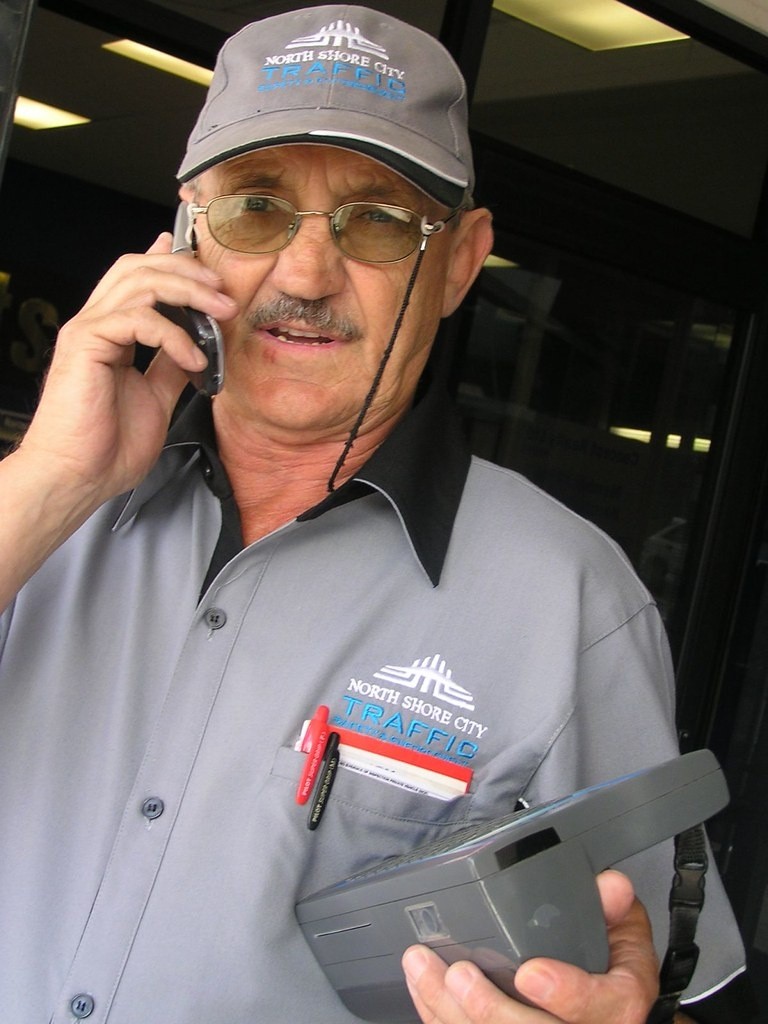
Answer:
[0,5,744,1023]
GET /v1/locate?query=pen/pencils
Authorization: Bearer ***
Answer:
[290,705,332,807]
[304,730,343,832]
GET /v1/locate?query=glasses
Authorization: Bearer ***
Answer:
[184,193,458,266]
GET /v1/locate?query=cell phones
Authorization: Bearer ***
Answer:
[159,200,224,397]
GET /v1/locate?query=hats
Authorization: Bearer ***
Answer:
[174,5,478,212]
